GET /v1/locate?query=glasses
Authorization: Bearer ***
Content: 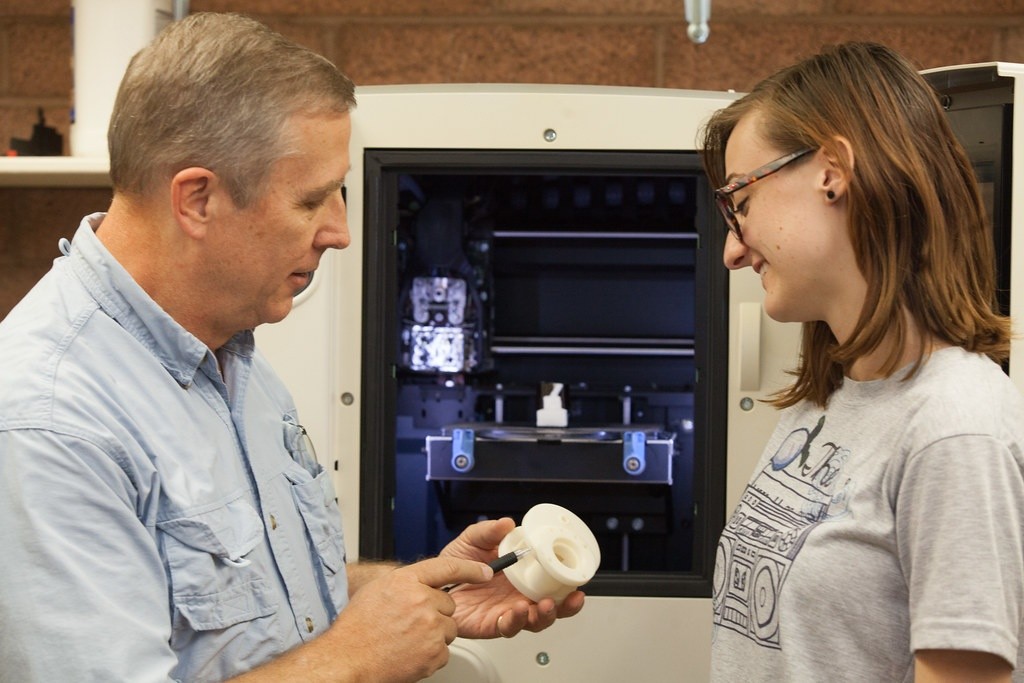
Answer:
[714,144,820,240]
[288,423,318,478]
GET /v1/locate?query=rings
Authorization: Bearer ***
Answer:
[497,616,510,639]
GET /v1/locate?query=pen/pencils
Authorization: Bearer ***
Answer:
[439,547,532,595]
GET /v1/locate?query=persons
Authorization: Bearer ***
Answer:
[0,10,586,683]
[708,40,1024,682]
